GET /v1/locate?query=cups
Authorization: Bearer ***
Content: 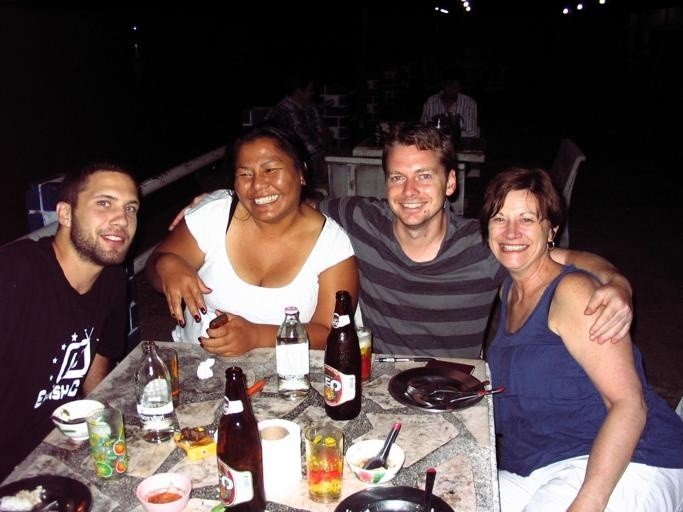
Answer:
[158,347,179,403]
[355,326,373,381]
[84,407,129,479]
[305,426,345,504]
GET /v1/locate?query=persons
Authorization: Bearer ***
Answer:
[165,116,634,360]
[143,123,360,363]
[477,162,682,511]
[262,71,333,181]
[415,72,480,141]
[0,153,142,480]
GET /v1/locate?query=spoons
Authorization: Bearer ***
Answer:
[365,422,402,468]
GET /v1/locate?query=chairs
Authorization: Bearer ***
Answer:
[551,139,586,247]
[317,147,471,222]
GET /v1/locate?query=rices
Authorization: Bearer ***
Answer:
[0,484,48,512]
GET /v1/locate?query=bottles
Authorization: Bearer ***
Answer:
[323,290,363,421]
[213,365,267,512]
[274,306,311,400]
[135,341,175,445]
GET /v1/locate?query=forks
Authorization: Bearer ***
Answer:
[405,379,488,406]
[403,381,505,397]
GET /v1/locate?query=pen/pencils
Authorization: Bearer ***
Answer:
[374,358,436,362]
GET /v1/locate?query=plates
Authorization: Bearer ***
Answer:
[0,472,93,511]
[388,366,487,412]
[330,483,456,511]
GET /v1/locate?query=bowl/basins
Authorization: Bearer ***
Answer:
[134,472,191,511]
[343,439,404,482]
[49,398,106,440]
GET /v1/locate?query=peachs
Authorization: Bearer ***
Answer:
[117,462,127,471]
[115,443,125,454]
